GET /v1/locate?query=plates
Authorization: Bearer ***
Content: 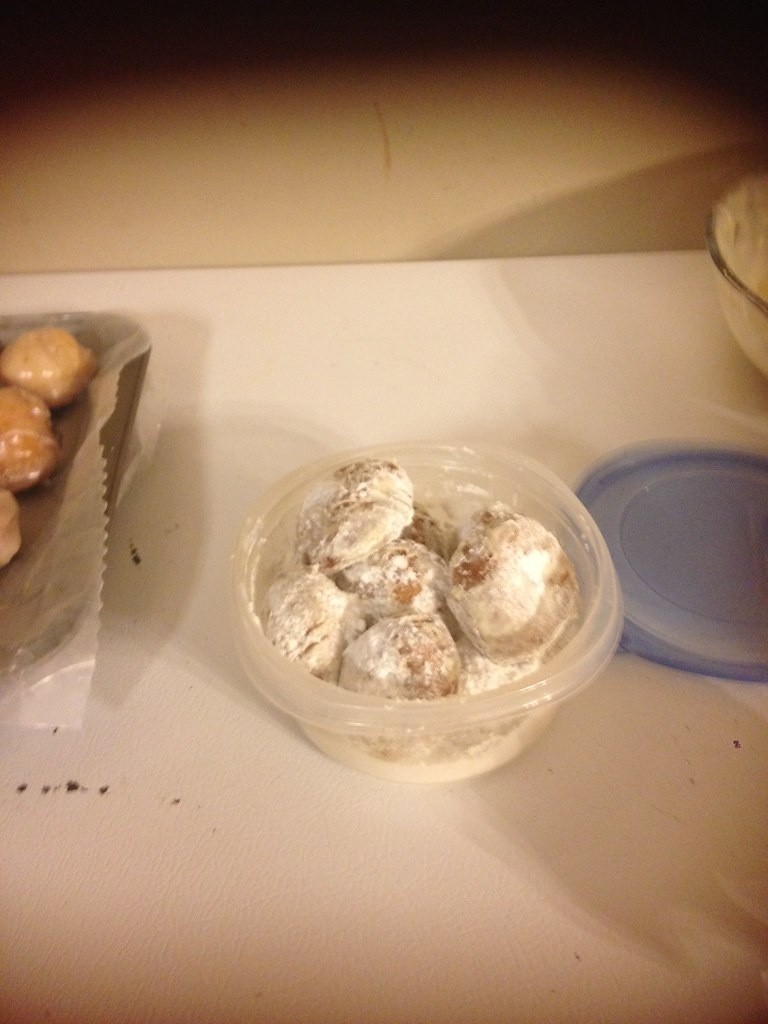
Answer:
[1,309,153,678]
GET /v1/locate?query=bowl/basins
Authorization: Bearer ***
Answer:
[703,148,768,378]
[229,437,628,788]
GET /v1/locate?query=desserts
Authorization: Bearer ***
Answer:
[259,455,582,776]
